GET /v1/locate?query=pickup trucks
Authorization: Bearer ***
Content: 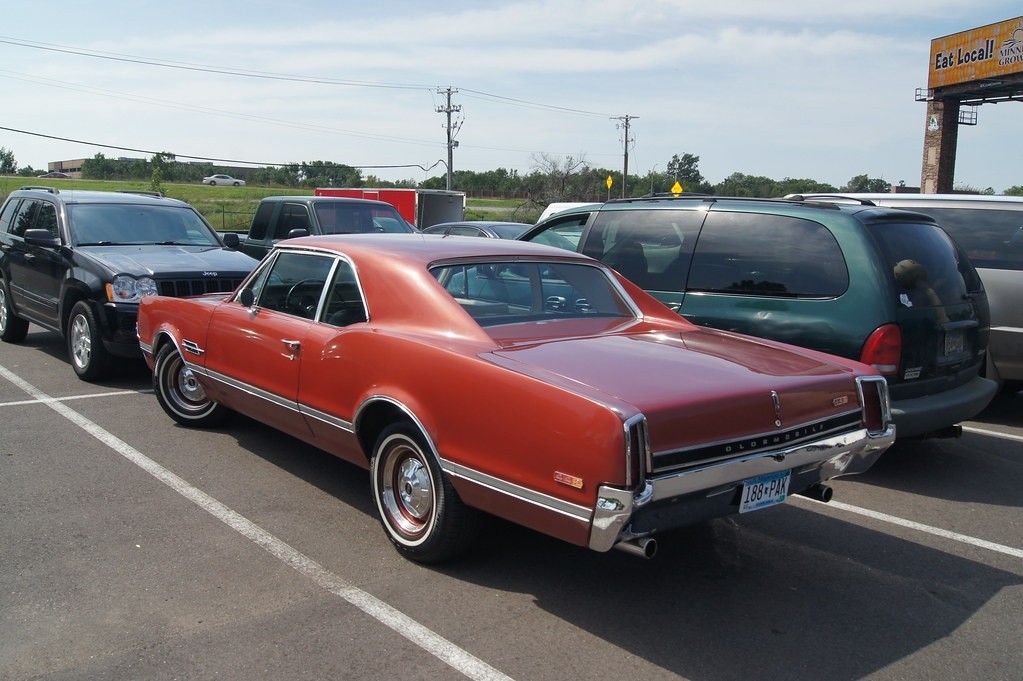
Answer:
[181,195,416,282]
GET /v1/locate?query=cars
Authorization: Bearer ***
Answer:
[418,219,584,314]
[202,174,246,187]
[37,171,71,180]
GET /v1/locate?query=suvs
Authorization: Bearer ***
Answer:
[0,185,285,383]
[434,188,1000,446]
[536,189,1023,393]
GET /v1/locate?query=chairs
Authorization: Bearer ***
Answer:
[602,231,714,290]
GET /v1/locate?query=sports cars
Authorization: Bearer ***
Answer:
[135,229,898,567]
